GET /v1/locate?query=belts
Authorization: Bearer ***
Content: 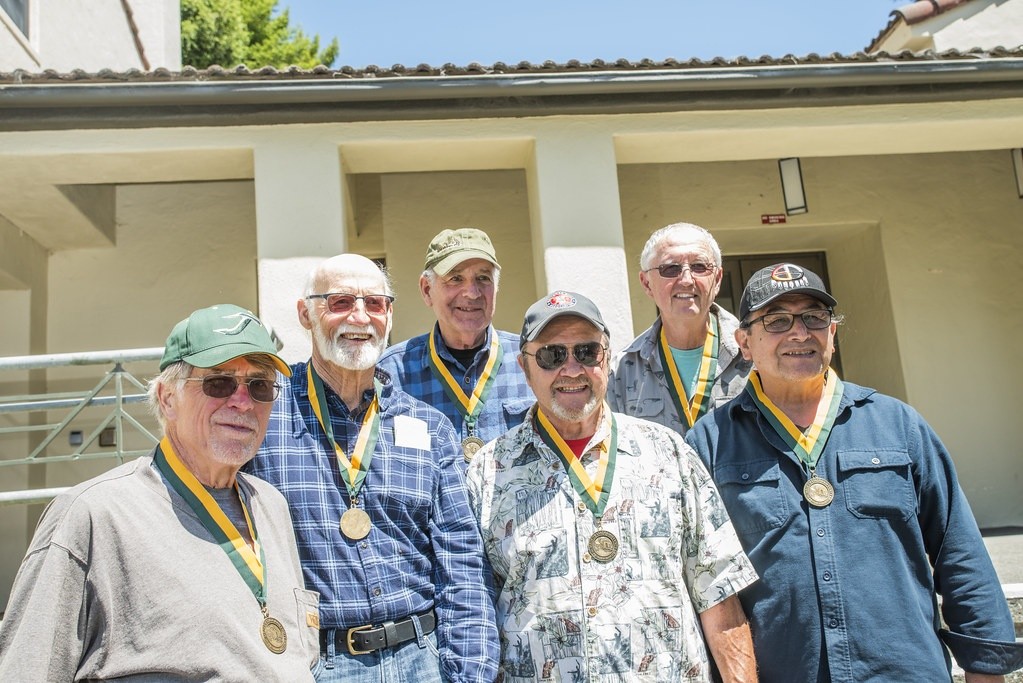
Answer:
[320,608,438,655]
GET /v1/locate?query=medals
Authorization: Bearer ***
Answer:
[340,508,371,539]
[804,477,834,507]
[462,437,485,463]
[588,530,619,562]
[261,618,288,654]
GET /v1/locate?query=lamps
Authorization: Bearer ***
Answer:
[1011,147,1023,199]
[778,156,810,217]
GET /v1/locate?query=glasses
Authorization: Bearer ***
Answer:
[524,342,607,369]
[187,375,282,403]
[746,309,832,333]
[305,292,394,314]
[646,262,718,279]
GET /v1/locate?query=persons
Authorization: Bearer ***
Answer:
[604,221,758,437]
[239,253,502,683]
[683,262,1023,683]
[376,228,538,475]
[466,290,760,683]
[0,304,320,683]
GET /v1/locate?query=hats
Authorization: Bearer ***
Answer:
[159,304,294,378]
[739,262,839,323]
[519,289,610,350]
[423,227,502,277]
